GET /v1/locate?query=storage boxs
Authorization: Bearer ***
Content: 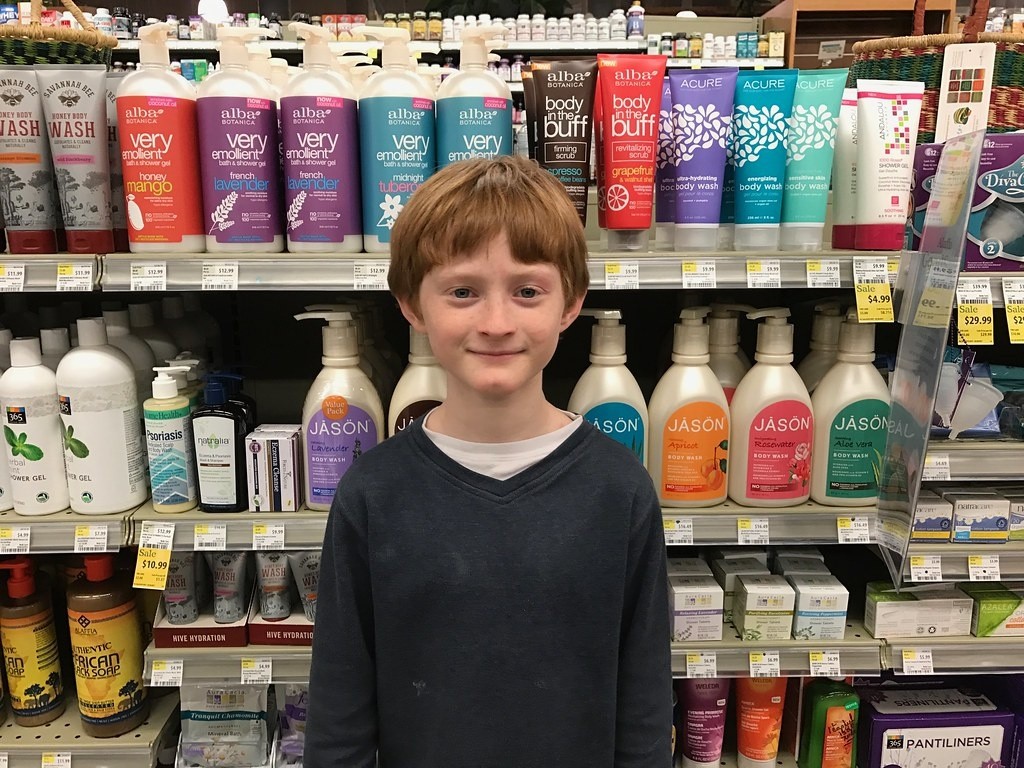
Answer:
[848,673,1024,767]
[908,132,1024,273]
[667,483,1024,642]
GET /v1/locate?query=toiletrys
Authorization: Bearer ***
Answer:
[0,1,1022,768]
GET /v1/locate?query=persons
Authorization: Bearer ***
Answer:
[300,153,674,768]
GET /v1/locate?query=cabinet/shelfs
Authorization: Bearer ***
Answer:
[0,41,1024,767]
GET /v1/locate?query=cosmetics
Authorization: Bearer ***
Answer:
[1,0,924,255]
